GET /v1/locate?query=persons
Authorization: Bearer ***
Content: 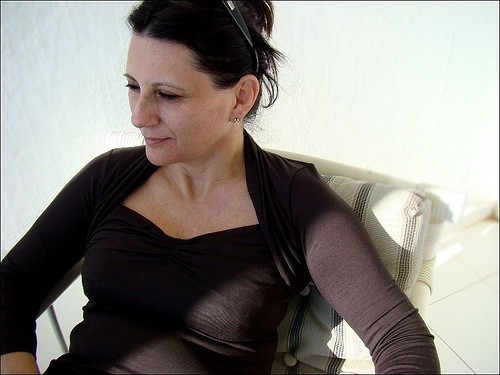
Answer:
[0,1,444,374]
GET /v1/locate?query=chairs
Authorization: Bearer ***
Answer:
[47,149,448,375]
